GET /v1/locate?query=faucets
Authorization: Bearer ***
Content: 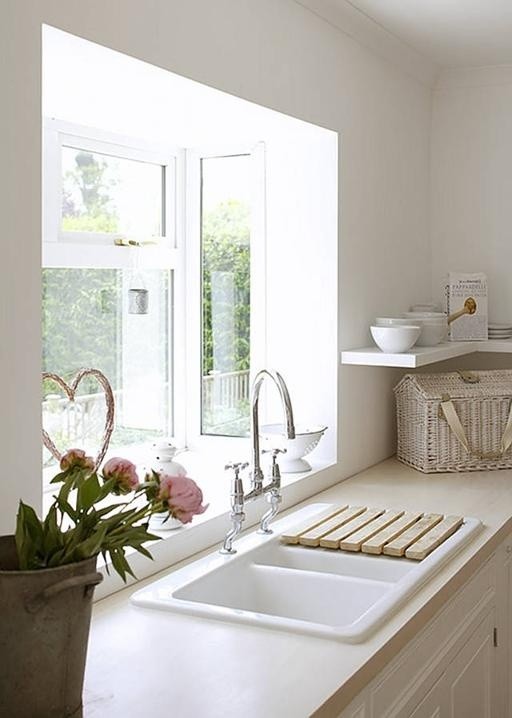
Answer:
[249,367,296,492]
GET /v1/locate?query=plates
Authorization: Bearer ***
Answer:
[486,323,512,339]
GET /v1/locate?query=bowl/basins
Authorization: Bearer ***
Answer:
[370,326,421,353]
[375,310,449,347]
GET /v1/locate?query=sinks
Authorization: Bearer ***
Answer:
[128,564,398,646]
[279,512,485,560]
[251,542,422,583]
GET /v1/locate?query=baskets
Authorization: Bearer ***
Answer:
[392,369,512,473]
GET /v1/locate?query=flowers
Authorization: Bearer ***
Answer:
[13,448,209,584]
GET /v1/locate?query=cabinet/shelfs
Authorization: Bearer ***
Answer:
[369,553,501,718]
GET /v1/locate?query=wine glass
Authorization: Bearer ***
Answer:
[258,423,328,473]
